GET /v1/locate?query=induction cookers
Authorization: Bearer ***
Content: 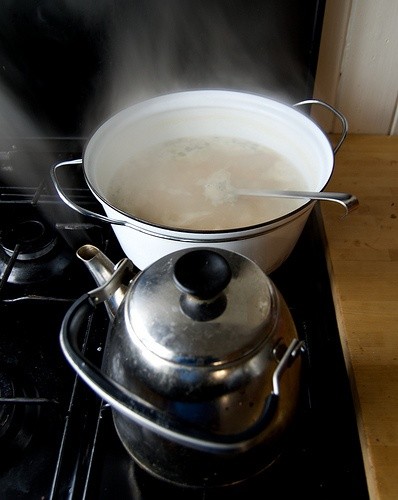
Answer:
[0,135,368,500]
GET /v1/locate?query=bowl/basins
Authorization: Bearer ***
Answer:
[52,89,348,275]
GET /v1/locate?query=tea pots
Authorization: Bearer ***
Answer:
[60,245,306,488]
